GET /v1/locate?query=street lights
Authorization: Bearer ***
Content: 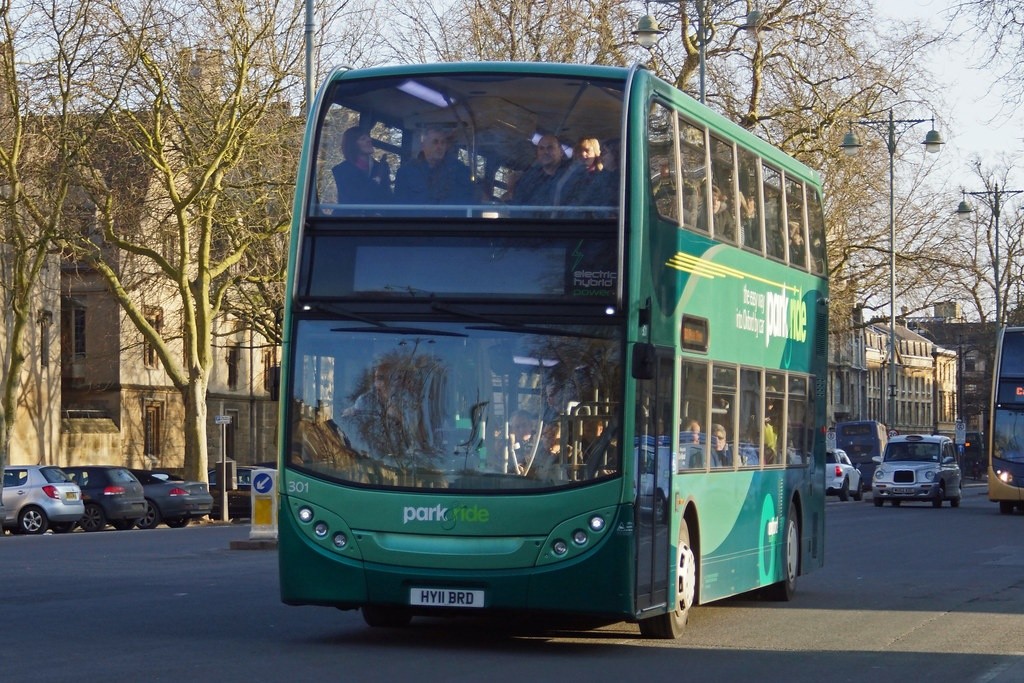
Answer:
[955,188,1023,346]
[838,109,942,424]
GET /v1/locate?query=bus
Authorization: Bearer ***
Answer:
[956,430,989,482]
[833,420,888,492]
[278,62,827,638]
[825,426,839,449]
[984,326,1024,517]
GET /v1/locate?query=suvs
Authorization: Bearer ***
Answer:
[809,446,864,501]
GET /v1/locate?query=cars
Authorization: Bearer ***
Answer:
[128,467,213,529]
[0,464,85,534]
[201,464,271,525]
[57,465,150,534]
[871,434,963,510]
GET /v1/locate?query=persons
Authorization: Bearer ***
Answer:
[559,140,624,223]
[393,127,476,220]
[649,152,827,274]
[333,127,399,218]
[429,372,809,484]
[569,136,606,174]
[342,365,424,463]
[510,134,571,219]
